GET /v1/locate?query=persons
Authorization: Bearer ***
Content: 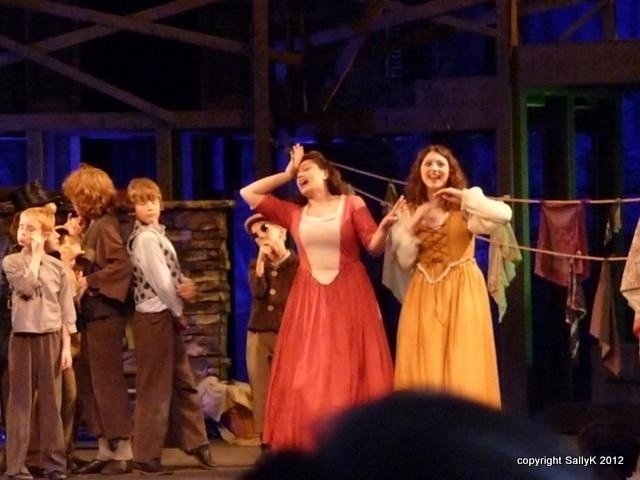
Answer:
[375,146,521,415]
[239,142,406,455]
[0,162,218,480]
[235,387,640,480]
[241,214,302,447]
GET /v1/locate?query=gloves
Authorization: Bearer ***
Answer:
[225,409,261,440]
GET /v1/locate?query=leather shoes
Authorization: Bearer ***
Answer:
[72,458,105,473]
[11,473,34,480]
[184,443,217,469]
[48,470,66,479]
[132,460,174,474]
[101,459,133,475]
[67,453,90,473]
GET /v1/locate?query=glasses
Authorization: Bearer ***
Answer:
[251,223,268,239]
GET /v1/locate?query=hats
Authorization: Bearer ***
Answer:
[243,213,266,233]
